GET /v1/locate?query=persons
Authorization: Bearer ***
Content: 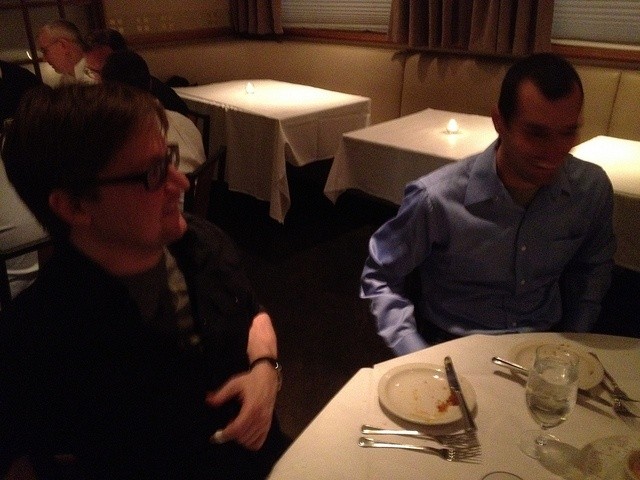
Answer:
[0,20,205,213]
[359,52,616,356]
[0,81,286,480]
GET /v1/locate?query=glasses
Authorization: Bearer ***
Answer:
[38,39,58,54]
[60,142,181,191]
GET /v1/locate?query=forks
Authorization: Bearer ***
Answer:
[359,424,481,462]
[588,352,640,419]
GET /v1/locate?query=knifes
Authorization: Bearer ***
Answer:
[445,355,476,427]
[491,357,614,408]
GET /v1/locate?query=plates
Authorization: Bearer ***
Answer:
[509,339,605,390]
[624,447,640,480]
[379,362,476,425]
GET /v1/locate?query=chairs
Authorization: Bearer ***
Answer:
[0,109,228,318]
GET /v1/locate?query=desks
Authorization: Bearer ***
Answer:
[267,335,640,480]
[324,107,499,208]
[569,134,640,274]
[172,76,372,224]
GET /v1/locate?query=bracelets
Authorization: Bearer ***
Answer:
[247,354,283,393]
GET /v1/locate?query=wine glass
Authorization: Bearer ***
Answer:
[518,343,579,461]
